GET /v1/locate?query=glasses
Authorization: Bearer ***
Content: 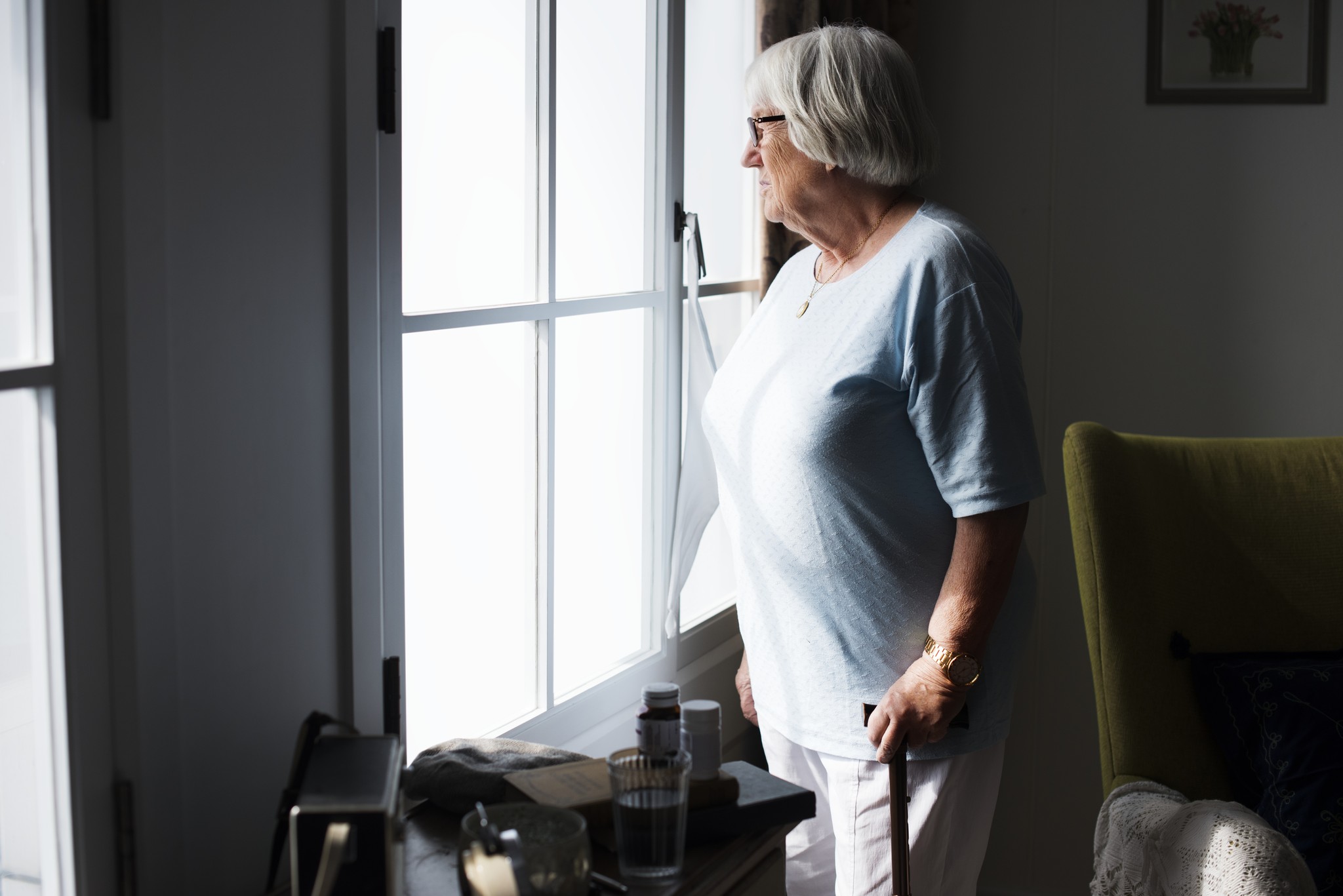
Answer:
[747,115,786,147]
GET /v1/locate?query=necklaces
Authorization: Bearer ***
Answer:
[796,199,900,320]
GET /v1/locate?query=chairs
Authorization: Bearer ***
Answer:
[1062,421,1343,896]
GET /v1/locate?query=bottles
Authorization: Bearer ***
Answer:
[634,682,683,768]
[679,699,722,781]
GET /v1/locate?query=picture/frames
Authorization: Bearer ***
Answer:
[1145,0,1330,105]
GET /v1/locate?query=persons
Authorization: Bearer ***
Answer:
[699,25,1045,896]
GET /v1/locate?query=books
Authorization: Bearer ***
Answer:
[501,758,815,847]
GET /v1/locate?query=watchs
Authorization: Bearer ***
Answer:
[926,637,982,687]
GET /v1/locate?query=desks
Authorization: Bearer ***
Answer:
[392,799,801,895]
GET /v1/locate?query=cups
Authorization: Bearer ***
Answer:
[456,803,591,896]
[604,745,693,876]
